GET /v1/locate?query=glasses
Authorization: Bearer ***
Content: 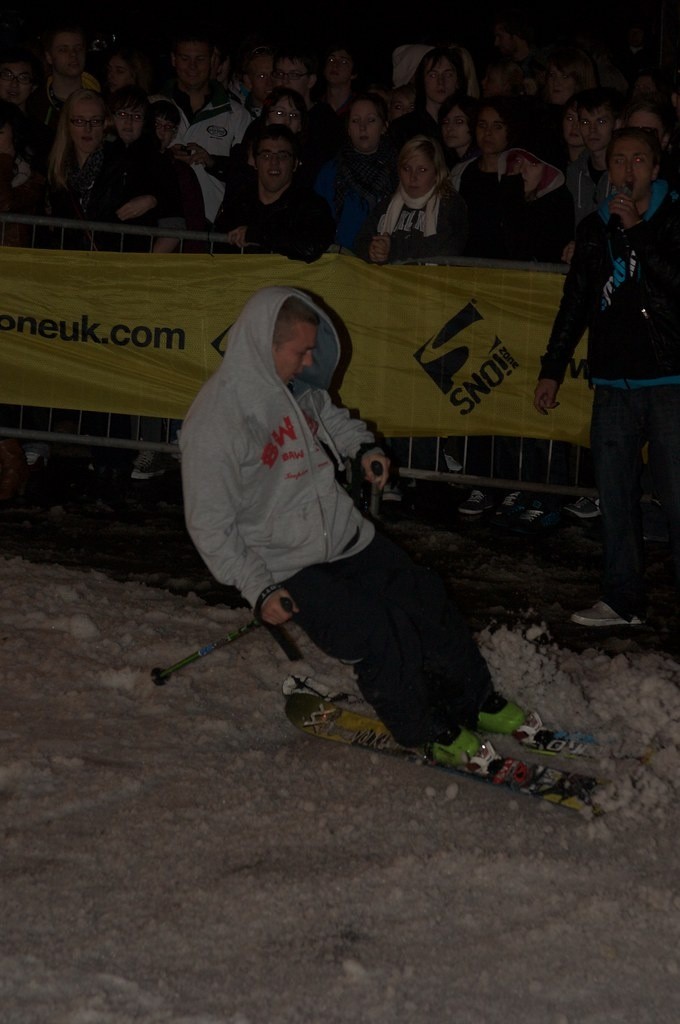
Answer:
[152,122,175,132]
[269,110,302,120]
[71,119,104,127]
[275,72,308,80]
[113,111,145,122]
[0,72,32,84]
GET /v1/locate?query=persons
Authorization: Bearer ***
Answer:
[1,21,680,521]
[177,285,550,784]
[535,126,680,627]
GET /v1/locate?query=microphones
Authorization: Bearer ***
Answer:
[604,181,633,238]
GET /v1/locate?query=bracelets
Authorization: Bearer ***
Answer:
[18,172,29,177]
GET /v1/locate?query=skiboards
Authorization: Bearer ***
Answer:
[281,673,653,817]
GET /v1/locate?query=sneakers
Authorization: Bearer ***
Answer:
[571,601,643,625]
[470,693,525,734]
[496,493,521,516]
[511,500,555,532]
[418,726,481,766]
[458,490,493,514]
[564,496,601,518]
[130,453,166,478]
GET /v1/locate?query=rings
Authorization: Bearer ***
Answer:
[619,204,621,208]
[620,198,624,204]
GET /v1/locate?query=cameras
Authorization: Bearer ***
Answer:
[181,146,196,156]
